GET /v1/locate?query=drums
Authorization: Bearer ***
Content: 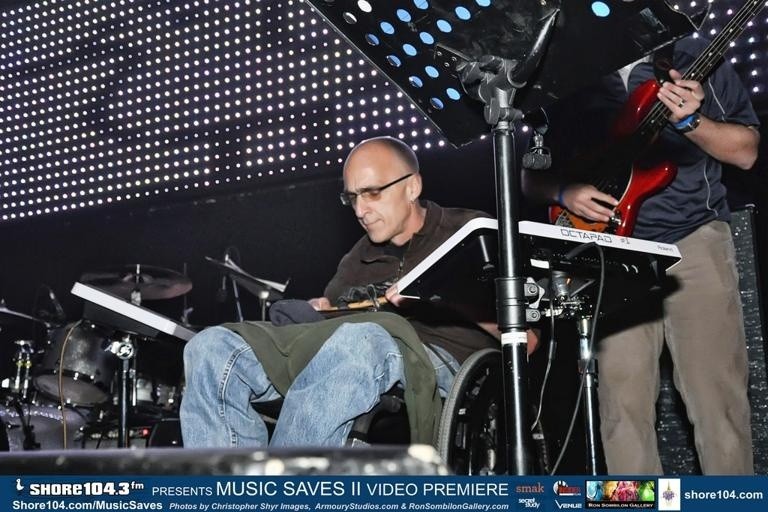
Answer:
[1,308,46,407]
[35,319,117,408]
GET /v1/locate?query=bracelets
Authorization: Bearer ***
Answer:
[673,115,701,134]
[557,181,565,208]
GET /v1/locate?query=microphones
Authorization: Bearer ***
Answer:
[366,286,380,310]
[522,133,552,170]
[217,253,229,302]
[49,288,66,321]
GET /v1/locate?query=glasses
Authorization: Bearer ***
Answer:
[340,173,414,206]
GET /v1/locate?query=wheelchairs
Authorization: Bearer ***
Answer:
[250,349,550,477]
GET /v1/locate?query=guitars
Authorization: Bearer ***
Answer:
[549,0,764,235]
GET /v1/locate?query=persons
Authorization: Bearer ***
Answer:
[177,135,542,449]
[520,35,761,469]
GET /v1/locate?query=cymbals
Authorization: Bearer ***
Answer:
[80,264,191,300]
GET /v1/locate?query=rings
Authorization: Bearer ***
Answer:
[679,97,686,108]
